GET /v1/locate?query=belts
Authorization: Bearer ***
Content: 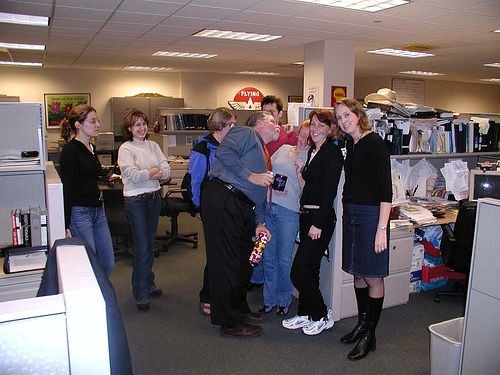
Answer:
[212,176,255,209]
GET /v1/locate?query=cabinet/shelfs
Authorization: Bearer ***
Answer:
[288,102,500,321]
[145,106,288,172]
[0,102,50,302]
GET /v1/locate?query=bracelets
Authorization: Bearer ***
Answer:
[377,225,386,230]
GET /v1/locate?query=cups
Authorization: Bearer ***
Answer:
[271,173,288,191]
[249,231,268,266]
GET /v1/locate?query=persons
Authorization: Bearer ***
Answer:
[282,109,344,336]
[181,107,238,316]
[258,119,310,321]
[59,104,115,278]
[335,98,393,360]
[117,110,171,311]
[200,111,280,337]
[247,95,298,291]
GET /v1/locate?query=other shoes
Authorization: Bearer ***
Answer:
[248,282,259,291]
[137,299,150,309]
[199,303,211,314]
[150,289,163,297]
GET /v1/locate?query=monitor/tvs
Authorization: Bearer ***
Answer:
[468,169,500,202]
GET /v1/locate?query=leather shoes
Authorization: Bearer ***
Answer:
[242,312,264,321]
[220,323,263,337]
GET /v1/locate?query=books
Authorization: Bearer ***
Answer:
[11,209,17,246]
[15,208,23,245]
[40,211,48,247]
[361,103,500,154]
[161,114,211,130]
[20,208,32,247]
[29,205,41,247]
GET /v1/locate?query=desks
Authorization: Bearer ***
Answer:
[97,176,178,190]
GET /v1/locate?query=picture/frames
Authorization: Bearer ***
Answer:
[44,93,92,130]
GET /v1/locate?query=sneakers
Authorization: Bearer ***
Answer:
[276,306,289,315]
[301,309,334,335]
[259,304,273,313]
[281,314,313,329]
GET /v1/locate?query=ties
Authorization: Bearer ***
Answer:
[263,145,273,210]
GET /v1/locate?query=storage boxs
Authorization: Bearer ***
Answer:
[95,132,115,151]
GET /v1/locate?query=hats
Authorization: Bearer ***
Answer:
[364,88,405,108]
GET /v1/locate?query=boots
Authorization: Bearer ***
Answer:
[347,296,384,360]
[340,287,368,344]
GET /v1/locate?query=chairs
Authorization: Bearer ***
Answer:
[433,202,477,304]
[101,190,133,257]
[160,173,199,251]
